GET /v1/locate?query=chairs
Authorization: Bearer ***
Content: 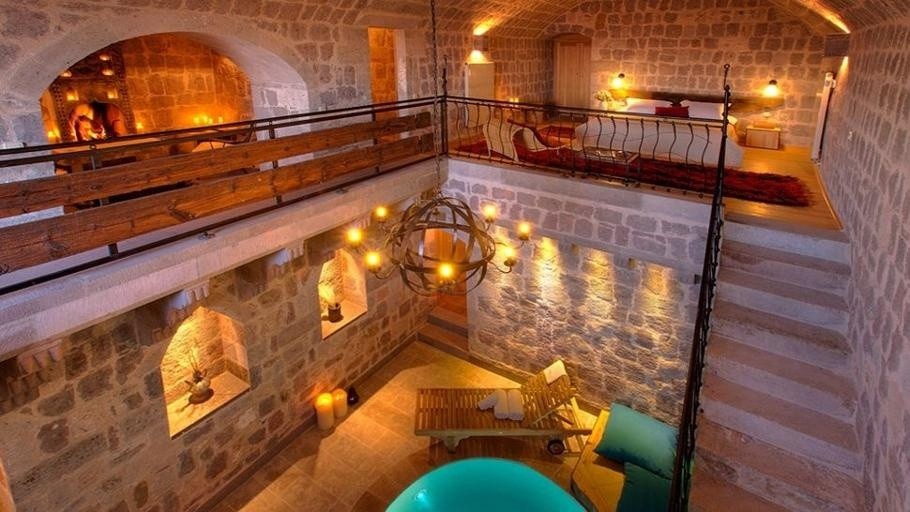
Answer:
[483,117,567,163]
[193,123,255,174]
[414,360,591,455]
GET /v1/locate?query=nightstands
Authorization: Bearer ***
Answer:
[747,123,781,150]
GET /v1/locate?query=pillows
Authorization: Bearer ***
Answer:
[617,462,672,512]
[593,402,679,479]
[624,96,731,120]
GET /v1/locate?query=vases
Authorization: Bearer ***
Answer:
[185,369,211,400]
[328,302,341,322]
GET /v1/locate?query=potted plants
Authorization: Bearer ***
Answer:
[594,91,611,110]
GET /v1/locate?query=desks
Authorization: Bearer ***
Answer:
[571,410,625,512]
[574,146,642,178]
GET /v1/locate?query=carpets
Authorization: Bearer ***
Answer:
[455,136,814,206]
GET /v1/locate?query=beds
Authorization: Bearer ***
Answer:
[575,90,745,180]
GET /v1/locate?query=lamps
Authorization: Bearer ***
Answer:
[767,80,778,95]
[315,393,334,431]
[825,33,849,56]
[473,35,489,51]
[614,74,625,84]
[349,0,531,296]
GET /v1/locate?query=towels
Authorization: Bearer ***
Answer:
[477,389,525,420]
[544,360,567,385]
